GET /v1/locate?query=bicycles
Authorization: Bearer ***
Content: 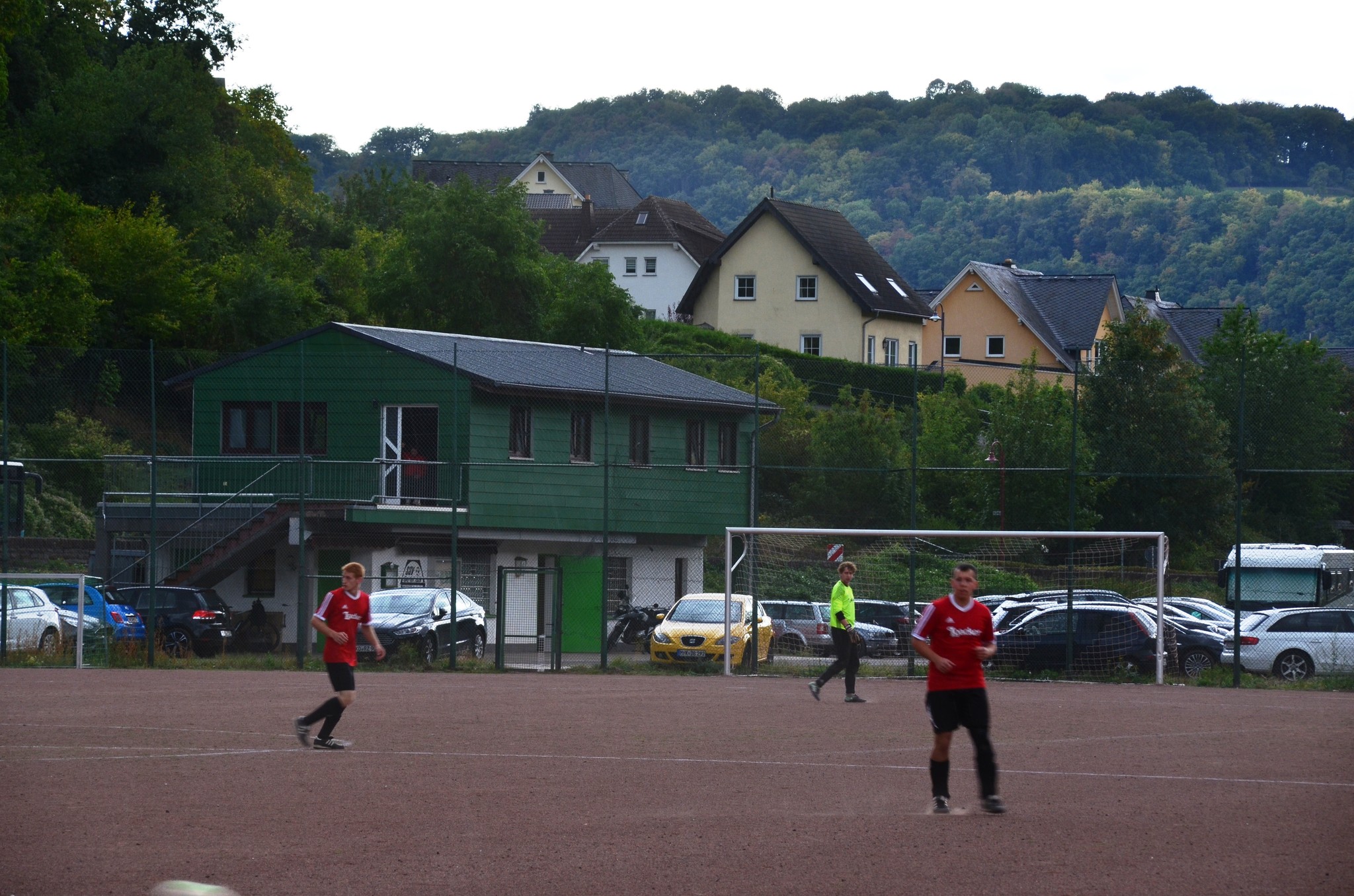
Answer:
[223,598,281,654]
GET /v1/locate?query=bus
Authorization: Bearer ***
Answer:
[1212,537,1352,627]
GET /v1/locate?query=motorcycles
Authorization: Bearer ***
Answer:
[607,583,670,654]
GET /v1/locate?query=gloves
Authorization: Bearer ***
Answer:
[844,624,860,643]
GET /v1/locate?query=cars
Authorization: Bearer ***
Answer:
[650,593,776,675]
[33,582,146,656]
[355,587,487,666]
[0,582,114,659]
[974,589,1245,681]
[854,620,898,659]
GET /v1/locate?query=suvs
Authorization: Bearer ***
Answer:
[1221,606,1354,684]
[854,599,931,658]
[119,585,235,659]
[759,599,834,658]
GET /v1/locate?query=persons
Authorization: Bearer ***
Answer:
[400,442,430,506]
[808,561,867,703]
[910,563,1010,814]
[294,562,385,748]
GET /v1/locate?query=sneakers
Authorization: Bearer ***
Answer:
[313,736,344,749]
[294,716,311,747]
[808,682,821,701]
[932,796,950,813]
[844,694,867,702]
[979,795,1009,814]
